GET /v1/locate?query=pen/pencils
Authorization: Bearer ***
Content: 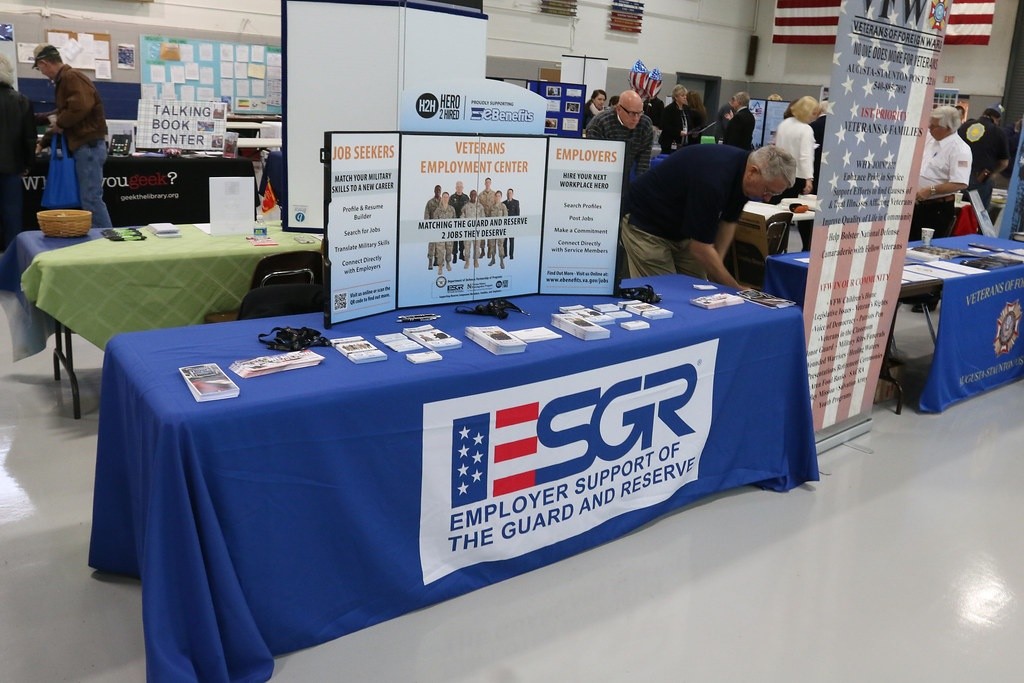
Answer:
[395,319,434,322]
[101,227,148,242]
[397,313,437,319]
[246,235,279,247]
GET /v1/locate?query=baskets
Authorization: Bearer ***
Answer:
[36,210,92,239]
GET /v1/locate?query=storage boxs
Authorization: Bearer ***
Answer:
[874,357,907,404]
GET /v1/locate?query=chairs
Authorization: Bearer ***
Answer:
[731,210,768,291]
[236,283,325,321]
[738,213,794,267]
[204,250,324,324]
[947,204,979,237]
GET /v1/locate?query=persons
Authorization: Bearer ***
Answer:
[644,85,707,155]
[586,91,654,184]
[769,96,829,253]
[900,106,972,312]
[32,43,113,228]
[714,92,757,149]
[584,89,619,128]
[0,54,38,252]
[420,332,448,341]
[620,144,796,291]
[955,105,1024,235]
[424,178,521,274]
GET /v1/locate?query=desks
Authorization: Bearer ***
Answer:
[761,234,1024,416]
[86,273,820,683]
[0,220,323,420]
[0,150,261,254]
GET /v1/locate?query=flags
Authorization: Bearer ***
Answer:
[262,183,276,214]
[771,0,996,45]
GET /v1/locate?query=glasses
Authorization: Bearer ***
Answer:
[753,163,781,197]
[618,104,645,117]
[928,124,945,131]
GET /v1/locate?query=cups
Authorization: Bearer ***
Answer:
[48,114,57,125]
[223,132,239,158]
[922,228,935,246]
[955,193,963,204]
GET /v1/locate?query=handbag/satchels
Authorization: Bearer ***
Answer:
[41,130,82,209]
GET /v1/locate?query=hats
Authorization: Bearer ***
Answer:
[984,103,1006,127]
[30,42,59,70]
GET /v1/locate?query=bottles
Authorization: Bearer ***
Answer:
[254,214,267,243]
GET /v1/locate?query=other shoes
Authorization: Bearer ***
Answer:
[911,300,938,314]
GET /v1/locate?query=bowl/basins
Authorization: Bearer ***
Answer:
[37,210,92,237]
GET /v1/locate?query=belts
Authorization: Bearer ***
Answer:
[935,194,955,202]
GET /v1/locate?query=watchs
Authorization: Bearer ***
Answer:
[931,185,936,196]
